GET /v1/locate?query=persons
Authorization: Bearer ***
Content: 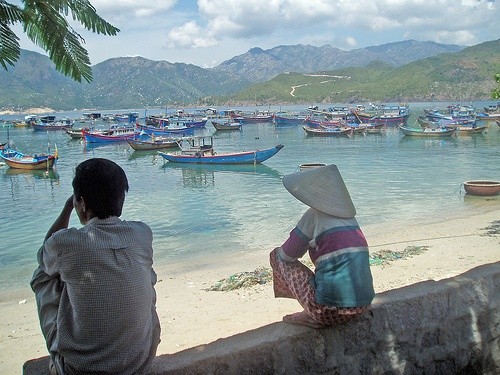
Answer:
[29,158,160,375]
[270,163,375,329]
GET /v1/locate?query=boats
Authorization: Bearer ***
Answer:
[298,163,327,172]
[158,135,285,165]
[0,100,500,151]
[0,143,59,171]
[125,132,193,151]
[463,179,500,196]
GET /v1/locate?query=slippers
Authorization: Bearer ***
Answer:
[283,314,323,329]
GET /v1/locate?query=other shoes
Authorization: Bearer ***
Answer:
[48,359,57,375]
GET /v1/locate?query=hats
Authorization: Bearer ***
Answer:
[283,164,356,219]
